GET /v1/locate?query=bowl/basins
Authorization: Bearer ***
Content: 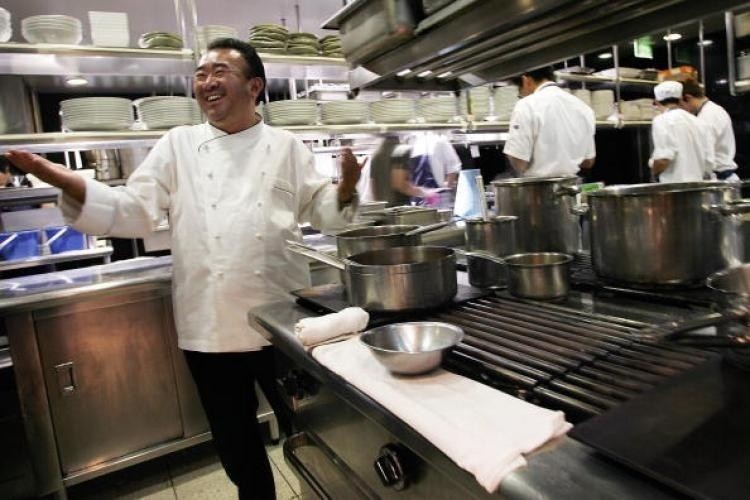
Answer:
[357,323,466,376]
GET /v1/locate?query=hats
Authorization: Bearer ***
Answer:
[653,80,685,102]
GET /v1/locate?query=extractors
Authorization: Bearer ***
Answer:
[320,1,750,82]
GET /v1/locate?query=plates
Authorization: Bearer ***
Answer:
[260,80,520,124]
[0,4,186,52]
[196,19,349,58]
[58,95,206,131]
[568,84,655,121]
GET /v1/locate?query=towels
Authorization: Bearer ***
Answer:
[295,304,371,354]
[310,331,575,494]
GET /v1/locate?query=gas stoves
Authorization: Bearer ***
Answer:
[249,259,748,499]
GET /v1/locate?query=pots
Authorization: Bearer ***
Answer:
[287,164,749,321]
[91,146,150,182]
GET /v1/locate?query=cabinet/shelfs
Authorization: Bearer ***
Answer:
[0,186,115,370]
[30,281,185,479]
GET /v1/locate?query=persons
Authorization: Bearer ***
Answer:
[370,133,440,207]
[502,64,597,181]
[647,81,716,182]
[6,38,368,500]
[391,131,463,210]
[679,77,740,184]
